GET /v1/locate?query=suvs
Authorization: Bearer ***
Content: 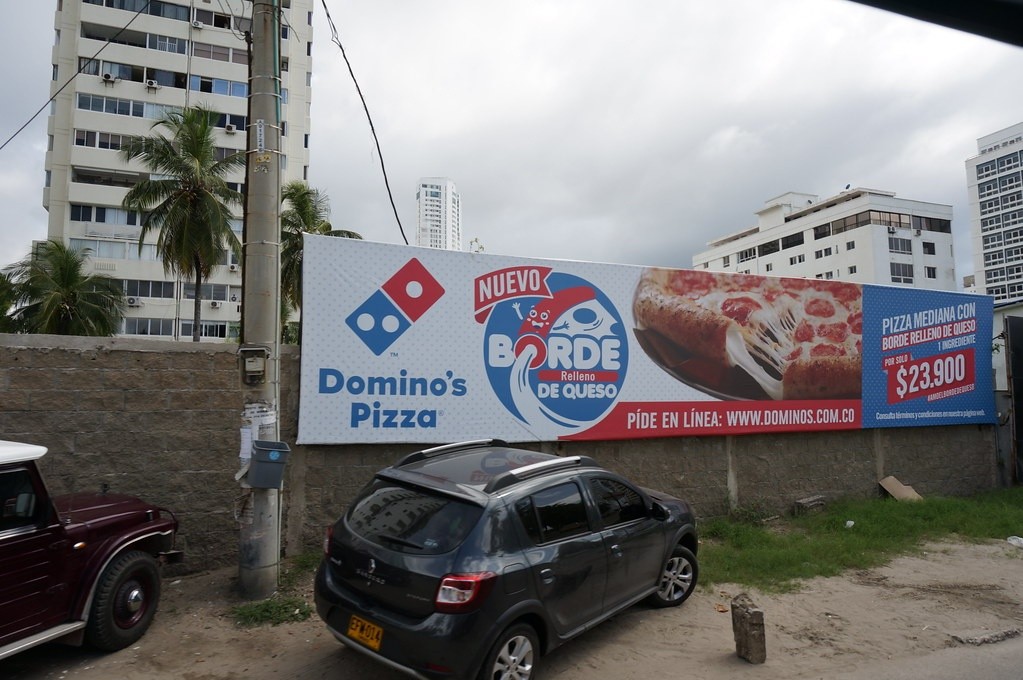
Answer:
[309,438,701,679]
[1,440,178,670]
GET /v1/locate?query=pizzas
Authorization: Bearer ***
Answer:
[634,268,863,400]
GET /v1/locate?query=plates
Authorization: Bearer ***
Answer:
[632,313,773,402]
